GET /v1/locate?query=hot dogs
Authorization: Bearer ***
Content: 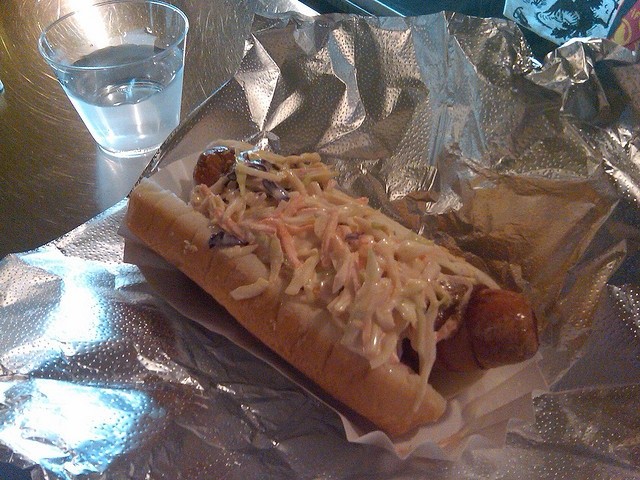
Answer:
[129,140,540,436]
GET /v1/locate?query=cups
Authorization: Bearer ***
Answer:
[39,1,189,160]
